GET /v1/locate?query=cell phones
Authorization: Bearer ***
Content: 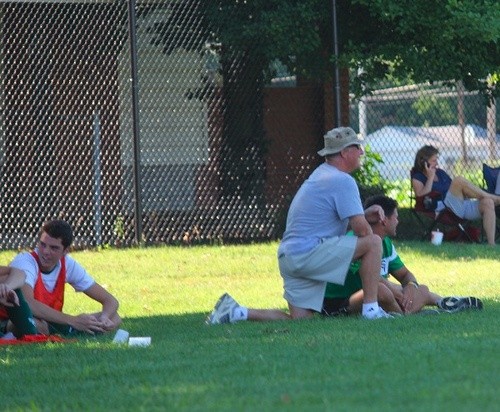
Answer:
[417,159,430,167]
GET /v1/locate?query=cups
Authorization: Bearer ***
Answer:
[431,231,443,244]
[129,337,152,346]
[112,329,129,345]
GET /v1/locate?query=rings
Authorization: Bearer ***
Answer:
[409,300,412,304]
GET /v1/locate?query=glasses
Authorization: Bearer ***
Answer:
[348,144,360,149]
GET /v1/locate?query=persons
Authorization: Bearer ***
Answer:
[0,265,50,337]
[205,128,402,325]
[7,221,122,336]
[410,145,500,244]
[320,194,483,317]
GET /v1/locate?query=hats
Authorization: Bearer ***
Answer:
[317,127,367,157]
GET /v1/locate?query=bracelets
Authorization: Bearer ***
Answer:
[403,281,418,288]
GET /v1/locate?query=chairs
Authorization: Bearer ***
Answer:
[407,168,481,243]
[479,162,500,196]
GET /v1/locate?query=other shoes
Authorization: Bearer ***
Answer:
[362,306,395,319]
[203,293,239,325]
[388,311,401,317]
[437,295,483,314]
